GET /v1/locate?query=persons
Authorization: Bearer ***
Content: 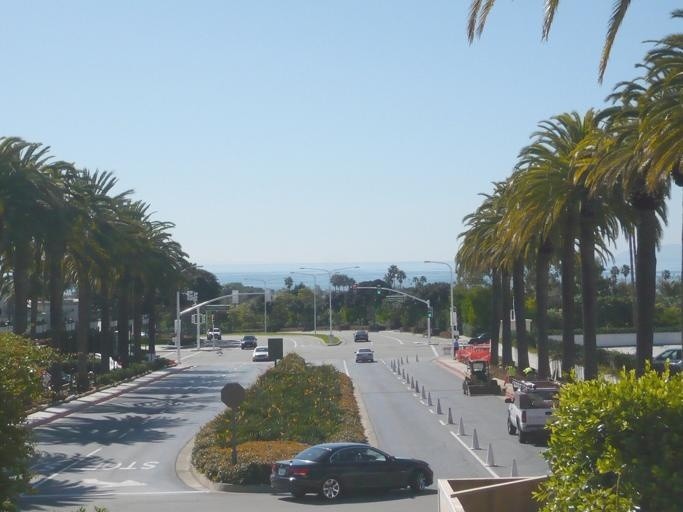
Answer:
[453,337,459,360]
[504,362,516,382]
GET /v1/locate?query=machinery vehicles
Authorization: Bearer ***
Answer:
[462,360,501,396]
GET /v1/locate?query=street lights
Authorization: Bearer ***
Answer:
[424,260,454,357]
[177,265,203,363]
[290,266,360,336]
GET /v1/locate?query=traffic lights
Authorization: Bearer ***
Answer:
[377,287,381,295]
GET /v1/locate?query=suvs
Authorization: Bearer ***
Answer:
[505,378,561,443]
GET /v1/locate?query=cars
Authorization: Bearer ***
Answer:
[271,443,432,501]
[653,347,683,367]
[253,346,268,361]
[352,329,368,342]
[240,336,256,349]
[468,333,489,344]
[207,328,221,339]
[354,348,374,362]
